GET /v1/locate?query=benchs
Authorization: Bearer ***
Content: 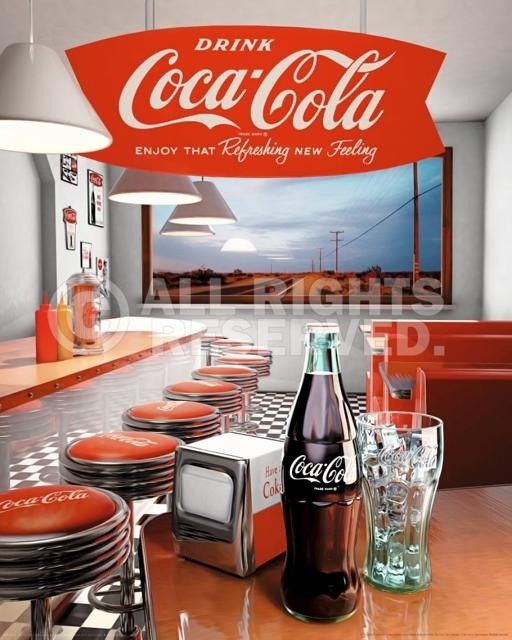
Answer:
[359,315,512,484]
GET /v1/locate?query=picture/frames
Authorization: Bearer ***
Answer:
[138,144,454,307]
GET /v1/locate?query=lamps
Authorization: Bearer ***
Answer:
[0,0,239,241]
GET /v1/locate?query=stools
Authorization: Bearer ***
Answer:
[0,333,274,638]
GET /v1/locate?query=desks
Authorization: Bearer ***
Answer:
[139,481,510,640]
